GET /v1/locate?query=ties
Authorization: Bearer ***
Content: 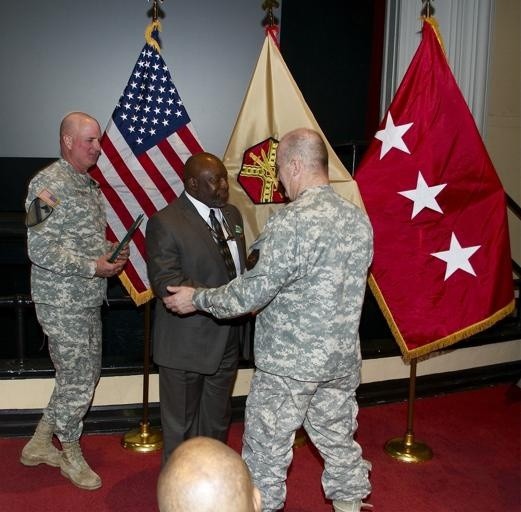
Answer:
[209,209,236,280]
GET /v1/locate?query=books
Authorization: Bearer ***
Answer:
[107,212,145,267]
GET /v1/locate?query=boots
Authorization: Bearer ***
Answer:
[60,441,102,490]
[20,414,62,468]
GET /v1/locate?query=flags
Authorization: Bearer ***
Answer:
[221,24,373,275]
[87,21,207,307]
[353,15,518,364]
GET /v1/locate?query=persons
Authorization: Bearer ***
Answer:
[156,434,264,512]
[18,110,130,492]
[161,128,377,512]
[145,149,251,461]
